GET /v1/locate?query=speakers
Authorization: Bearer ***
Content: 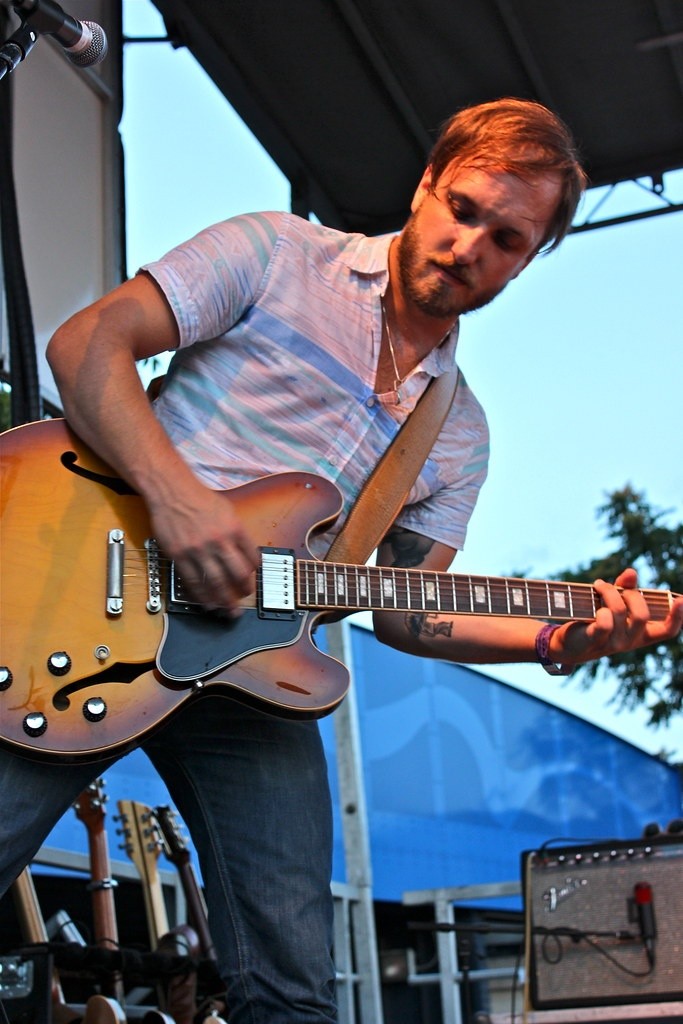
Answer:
[520,834,683,1008]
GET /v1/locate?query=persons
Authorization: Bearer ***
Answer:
[0,99,587,1024]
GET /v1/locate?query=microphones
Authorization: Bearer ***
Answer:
[633,880,657,965]
[10,0,108,68]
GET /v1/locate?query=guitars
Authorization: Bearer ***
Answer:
[112,796,229,1024]
[155,802,212,946]
[0,862,126,1022]
[61,766,179,1024]
[0,419,682,757]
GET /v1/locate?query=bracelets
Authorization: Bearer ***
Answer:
[535,622,577,676]
